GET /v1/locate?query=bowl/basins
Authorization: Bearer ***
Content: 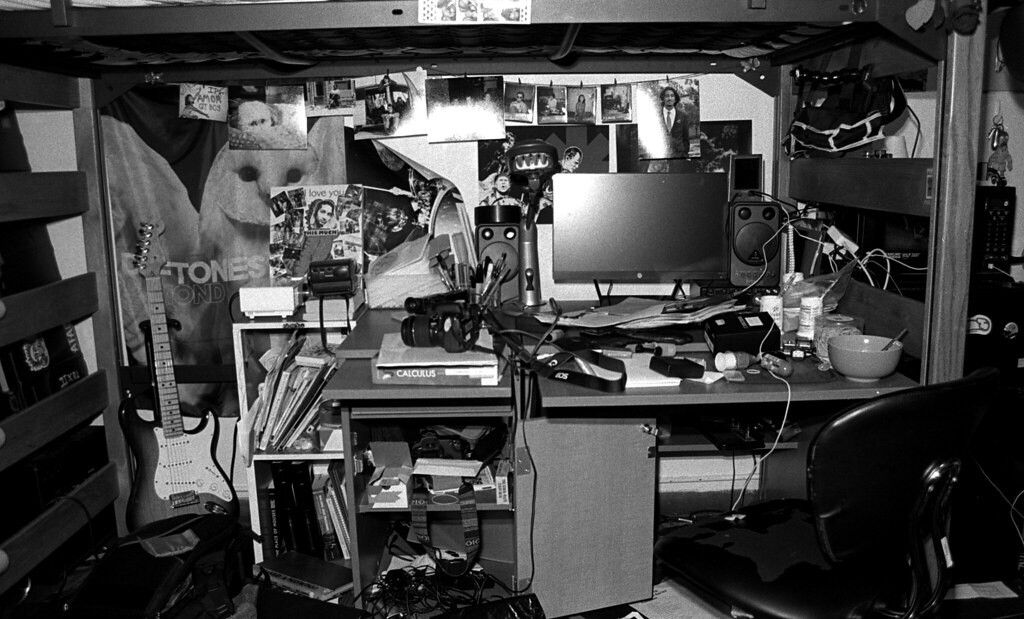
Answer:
[827,335,904,382]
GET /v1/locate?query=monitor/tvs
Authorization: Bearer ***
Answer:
[551,171,728,284]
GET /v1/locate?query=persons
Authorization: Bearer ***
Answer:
[326,84,340,109]
[648,87,690,158]
[988,131,1012,186]
[453,94,589,122]
[179,94,210,120]
[366,95,407,135]
[268,188,362,279]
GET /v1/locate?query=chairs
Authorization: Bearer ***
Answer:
[649,369,1024,619]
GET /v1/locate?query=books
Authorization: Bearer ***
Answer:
[237,330,341,468]
[573,350,682,388]
[370,330,499,386]
[253,461,351,606]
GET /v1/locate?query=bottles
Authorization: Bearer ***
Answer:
[815,312,865,372]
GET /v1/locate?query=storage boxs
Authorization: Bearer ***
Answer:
[366,441,414,509]
[495,460,510,505]
[305,289,365,313]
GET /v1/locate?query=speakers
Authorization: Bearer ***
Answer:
[476,223,520,308]
[730,201,781,287]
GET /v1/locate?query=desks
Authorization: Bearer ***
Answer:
[521,332,921,408]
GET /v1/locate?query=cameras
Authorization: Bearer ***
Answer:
[401,303,480,353]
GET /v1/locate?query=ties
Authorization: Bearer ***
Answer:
[667,112,671,133]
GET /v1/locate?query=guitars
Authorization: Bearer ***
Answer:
[117,215,241,538]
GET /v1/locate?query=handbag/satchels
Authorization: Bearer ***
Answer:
[64,515,255,619]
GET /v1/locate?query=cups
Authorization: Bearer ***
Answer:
[794,297,825,341]
[760,296,785,336]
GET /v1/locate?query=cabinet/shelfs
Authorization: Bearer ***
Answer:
[231,305,368,609]
[322,310,658,619]
